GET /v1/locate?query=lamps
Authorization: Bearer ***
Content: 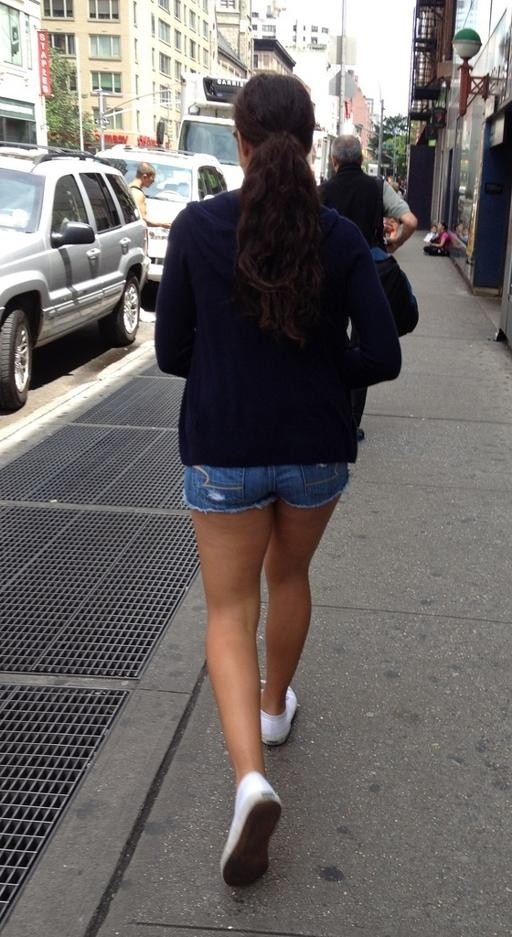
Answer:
[453,28,491,117]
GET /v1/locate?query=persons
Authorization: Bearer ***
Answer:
[424,222,450,256]
[317,135,419,439]
[126,161,172,230]
[454,223,468,243]
[423,225,437,245]
[379,173,407,240]
[153,74,401,889]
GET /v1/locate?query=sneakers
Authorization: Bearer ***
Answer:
[260,684,298,746]
[220,770,283,886]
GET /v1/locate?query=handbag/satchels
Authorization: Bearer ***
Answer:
[365,244,419,336]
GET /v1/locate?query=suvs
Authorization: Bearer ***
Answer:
[0,138,152,418]
[91,142,229,284]
